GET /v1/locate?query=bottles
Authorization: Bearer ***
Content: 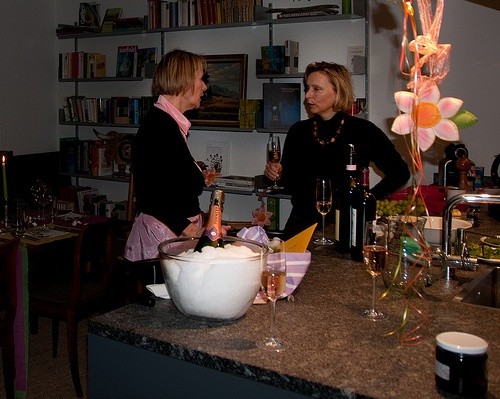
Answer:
[192,190,225,253]
[382,215,432,295]
[351,167,377,262]
[333,143,358,254]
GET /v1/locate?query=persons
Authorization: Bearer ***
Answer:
[267,61,409,247]
[122,51,219,309]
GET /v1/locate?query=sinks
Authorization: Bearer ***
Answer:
[452,265,500,311]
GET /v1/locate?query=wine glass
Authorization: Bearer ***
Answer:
[255,239,289,353]
[312,179,334,246]
[267,136,284,189]
[362,221,390,321]
[30,179,53,228]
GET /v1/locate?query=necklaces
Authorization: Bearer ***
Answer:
[311,115,348,145]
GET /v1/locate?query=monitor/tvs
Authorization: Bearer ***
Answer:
[8,152,71,206]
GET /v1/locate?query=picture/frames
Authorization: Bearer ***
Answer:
[183,53,248,125]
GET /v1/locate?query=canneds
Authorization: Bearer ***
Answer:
[434,331,488,399]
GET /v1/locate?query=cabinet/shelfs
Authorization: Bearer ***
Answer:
[57,1,371,200]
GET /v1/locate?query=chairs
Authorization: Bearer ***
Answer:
[28,217,119,396]
[0,236,20,398]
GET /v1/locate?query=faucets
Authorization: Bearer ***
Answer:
[442,193,500,271]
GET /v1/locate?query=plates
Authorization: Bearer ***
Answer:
[480,235,500,248]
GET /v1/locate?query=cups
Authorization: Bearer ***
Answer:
[471,166,485,194]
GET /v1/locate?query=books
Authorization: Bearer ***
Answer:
[57,45,157,234]
[213,175,255,193]
[55,0,263,36]
[236,37,302,132]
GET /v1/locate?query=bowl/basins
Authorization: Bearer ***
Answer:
[421,216,473,244]
[157,234,272,322]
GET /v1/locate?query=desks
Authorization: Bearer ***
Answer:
[0,226,79,252]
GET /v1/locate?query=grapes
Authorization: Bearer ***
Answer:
[376,195,425,217]
